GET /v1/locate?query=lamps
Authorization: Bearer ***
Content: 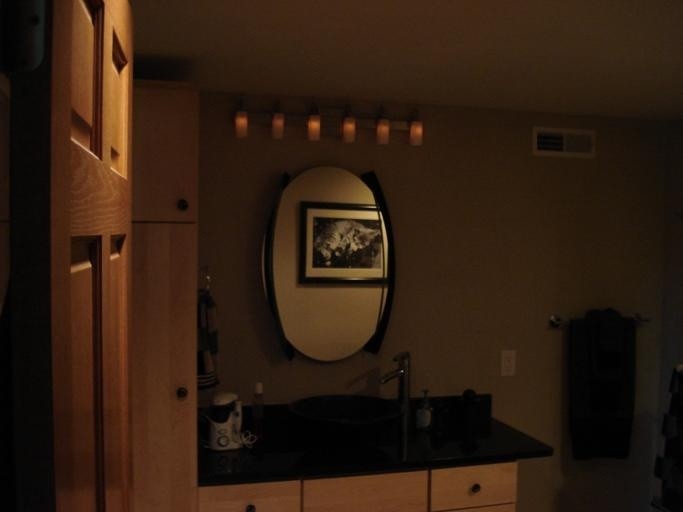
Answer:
[230,105,424,147]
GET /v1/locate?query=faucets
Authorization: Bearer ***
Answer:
[380,350,411,441]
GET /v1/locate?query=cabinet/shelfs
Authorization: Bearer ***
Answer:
[128,222,199,512]
[301,469,430,512]
[133,76,201,222]
[199,479,301,512]
[429,460,519,512]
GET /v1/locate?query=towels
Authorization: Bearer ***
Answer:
[648,362,683,511]
[564,307,636,460]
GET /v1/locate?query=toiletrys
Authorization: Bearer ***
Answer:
[251,380,264,431]
[416,387,435,432]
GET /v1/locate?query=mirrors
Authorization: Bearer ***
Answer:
[259,164,395,362]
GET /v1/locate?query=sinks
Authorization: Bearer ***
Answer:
[288,391,398,453]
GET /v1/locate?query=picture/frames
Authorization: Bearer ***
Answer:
[298,200,388,284]
